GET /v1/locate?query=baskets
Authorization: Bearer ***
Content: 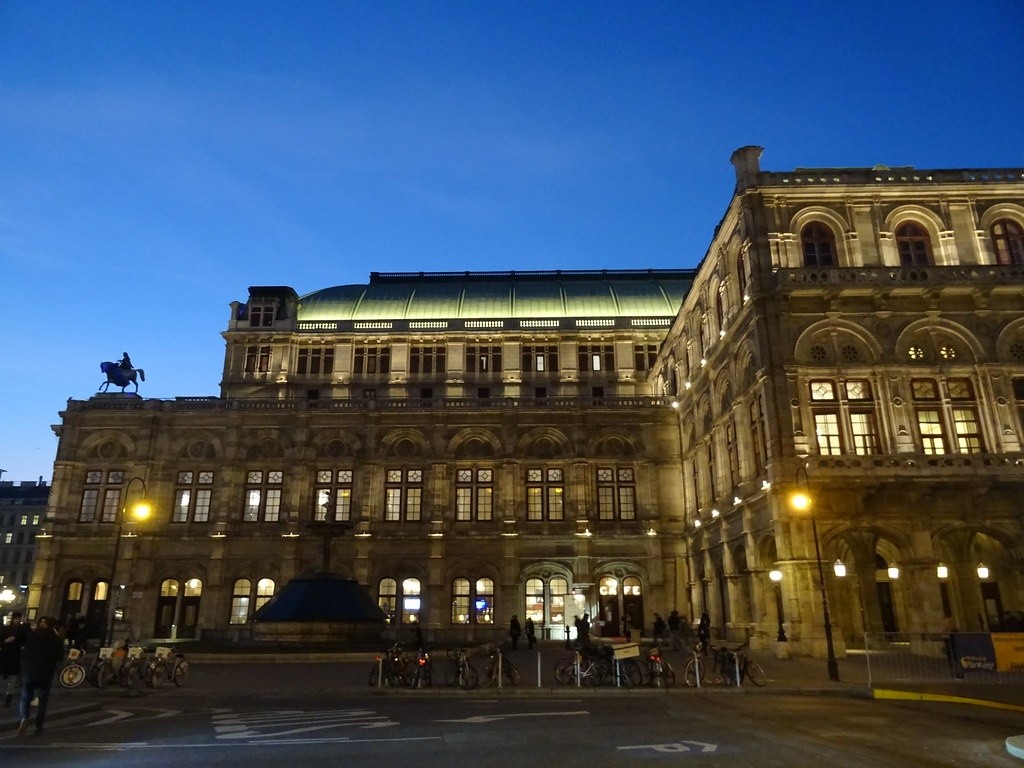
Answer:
[155,646,173,663]
[68,648,83,661]
[127,647,144,660]
[99,647,116,661]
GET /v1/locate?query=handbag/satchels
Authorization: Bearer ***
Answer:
[511,626,519,636]
[533,636,537,643]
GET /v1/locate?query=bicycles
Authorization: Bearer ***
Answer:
[58,641,191,690]
[368,639,524,690]
[684,641,769,689]
[554,643,676,690]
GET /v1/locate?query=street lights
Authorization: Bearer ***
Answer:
[788,467,842,684]
[98,476,152,648]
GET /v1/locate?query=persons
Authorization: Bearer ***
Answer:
[116,351,135,374]
[0,599,108,746]
[983,608,1024,635]
[509,602,722,655]
[409,619,425,662]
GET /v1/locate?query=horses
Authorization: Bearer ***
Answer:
[99,362,145,393]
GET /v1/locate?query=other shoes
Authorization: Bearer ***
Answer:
[626,640,631,643]
[33,723,42,736]
[674,649,680,651]
[17,717,29,738]
